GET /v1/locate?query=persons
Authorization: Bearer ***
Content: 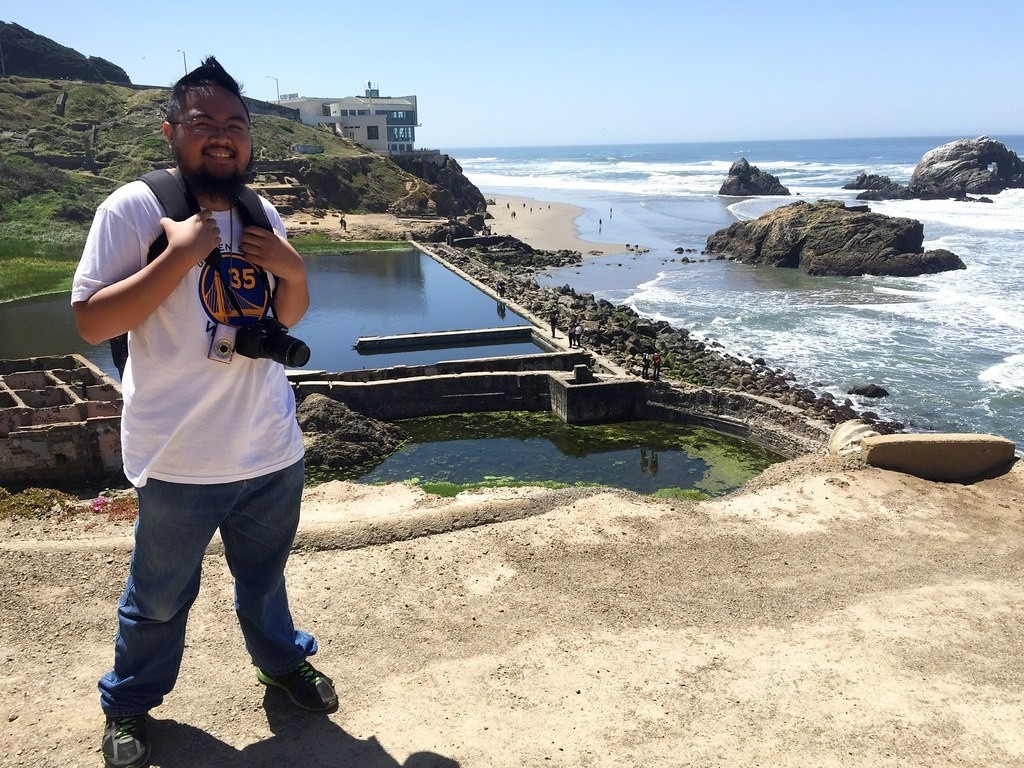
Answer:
[496,280,505,298]
[70,55,339,768]
[511,212,516,218]
[568,323,582,348]
[551,316,557,338]
[340,217,346,230]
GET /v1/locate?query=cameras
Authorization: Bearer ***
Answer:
[207,323,240,364]
[234,319,309,370]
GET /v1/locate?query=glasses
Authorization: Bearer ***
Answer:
[169,116,250,141]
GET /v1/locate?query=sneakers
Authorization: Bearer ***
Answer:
[253,656,338,711]
[100,714,152,768]
